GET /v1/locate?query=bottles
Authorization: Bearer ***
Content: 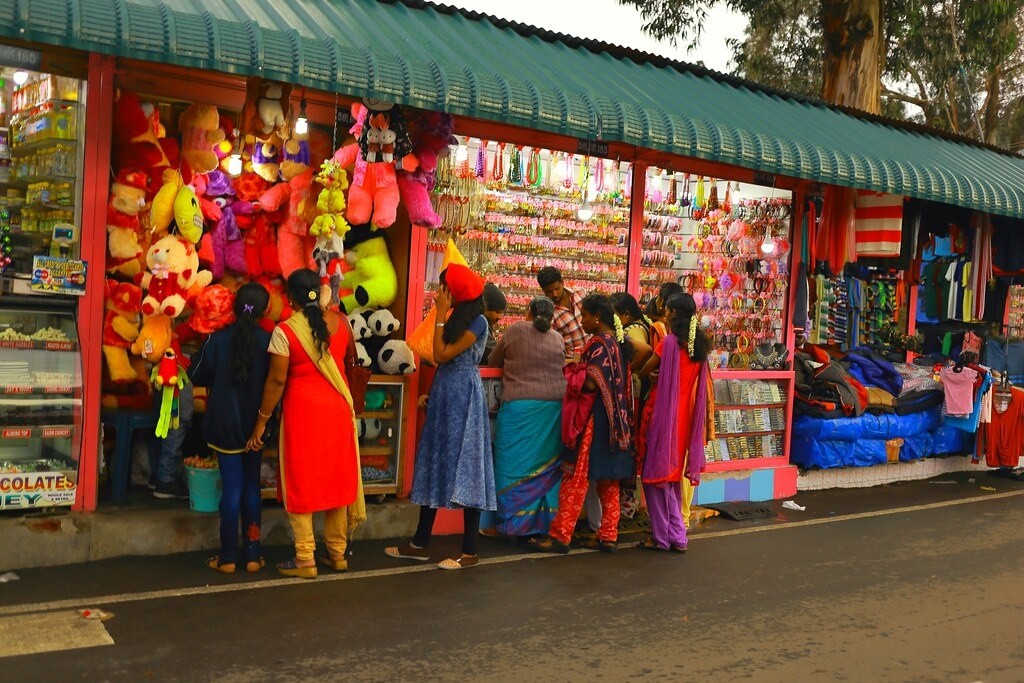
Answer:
[0,136,8,166]
[991,285,1024,343]
[0,79,7,127]
[9,70,81,274]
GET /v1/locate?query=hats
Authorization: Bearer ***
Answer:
[482,282,506,310]
[445,262,484,300]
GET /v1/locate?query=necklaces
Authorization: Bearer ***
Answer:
[423,141,792,370]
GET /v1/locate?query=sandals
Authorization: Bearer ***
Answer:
[204,555,235,573]
[637,538,670,551]
[577,538,618,552]
[527,538,569,553]
[246,558,265,571]
[670,544,687,552]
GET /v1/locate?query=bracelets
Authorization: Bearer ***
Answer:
[435,323,446,327]
[259,409,272,418]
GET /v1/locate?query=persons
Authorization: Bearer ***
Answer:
[478,282,506,368]
[147,310,194,499]
[527,282,715,554]
[384,263,497,569]
[537,266,594,359]
[185,282,281,576]
[479,297,568,548]
[246,267,367,578]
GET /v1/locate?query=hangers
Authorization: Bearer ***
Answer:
[932,253,972,263]
[996,373,1012,398]
[1010,273,1024,286]
[955,351,979,367]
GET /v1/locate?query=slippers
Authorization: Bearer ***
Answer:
[275,560,318,578]
[318,554,348,571]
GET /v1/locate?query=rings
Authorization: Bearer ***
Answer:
[249,441,252,444]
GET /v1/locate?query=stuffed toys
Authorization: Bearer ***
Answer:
[100,74,459,489]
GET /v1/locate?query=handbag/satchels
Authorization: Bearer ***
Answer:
[334,311,371,414]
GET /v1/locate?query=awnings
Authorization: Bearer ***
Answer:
[0,0,1024,219]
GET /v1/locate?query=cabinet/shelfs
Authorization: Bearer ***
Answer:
[700,370,795,473]
[359,372,410,498]
[0,75,81,252]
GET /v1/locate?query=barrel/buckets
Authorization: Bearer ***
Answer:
[184,464,220,512]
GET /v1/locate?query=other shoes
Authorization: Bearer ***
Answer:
[153,482,189,499]
[147,478,157,489]
[384,542,431,560]
[438,553,480,569]
